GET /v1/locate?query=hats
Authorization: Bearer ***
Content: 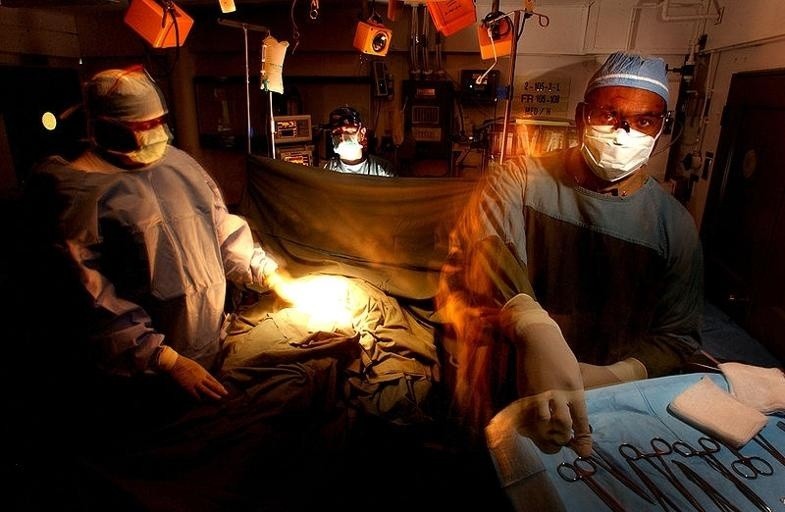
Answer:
[584,49,671,112]
[81,66,172,124]
[328,106,365,131]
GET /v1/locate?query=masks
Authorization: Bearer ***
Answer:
[331,140,364,162]
[575,104,666,184]
[117,123,170,169]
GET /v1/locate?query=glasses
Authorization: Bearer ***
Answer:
[581,107,670,134]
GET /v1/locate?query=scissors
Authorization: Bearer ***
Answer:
[558,422,785,512]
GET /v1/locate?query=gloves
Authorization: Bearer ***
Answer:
[496,290,594,460]
[156,345,230,403]
[576,355,649,393]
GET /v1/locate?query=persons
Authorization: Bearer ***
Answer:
[16,61,313,408]
[317,103,402,178]
[424,46,709,510]
